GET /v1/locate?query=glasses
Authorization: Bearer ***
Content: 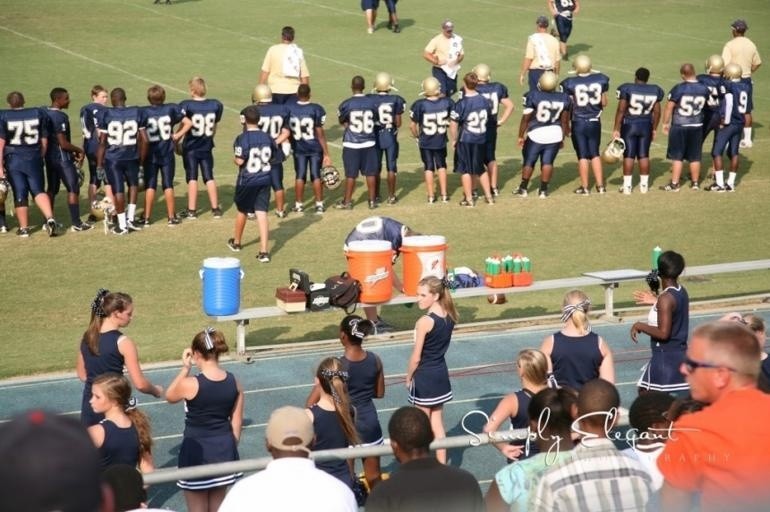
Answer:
[684,357,737,374]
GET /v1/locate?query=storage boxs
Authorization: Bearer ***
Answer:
[275,283,306,313]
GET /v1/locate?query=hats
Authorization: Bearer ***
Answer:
[265,405,314,455]
[536,16,549,28]
[442,21,456,31]
[731,19,748,32]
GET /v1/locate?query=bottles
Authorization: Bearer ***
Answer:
[652,245,662,273]
[485,256,533,275]
[448,267,456,294]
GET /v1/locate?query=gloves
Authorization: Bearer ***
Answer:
[138,166,144,185]
[97,167,106,180]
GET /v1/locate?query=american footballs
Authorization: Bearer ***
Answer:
[488,294,507,303]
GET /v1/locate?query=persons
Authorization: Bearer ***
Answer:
[366,72,407,204]
[227,106,287,262]
[724,313,770,394]
[548,0,581,61]
[450,73,496,206]
[304,356,372,511]
[97,88,149,235]
[85,371,154,474]
[333,76,380,210]
[657,320,770,512]
[560,56,610,194]
[660,64,713,192]
[365,406,488,511]
[288,84,332,214]
[260,26,310,106]
[687,54,725,180]
[134,84,193,228]
[80,84,109,208]
[405,276,458,465]
[512,71,571,199]
[613,67,665,194]
[647,398,702,511]
[540,290,614,393]
[40,87,97,232]
[483,348,553,464]
[218,405,359,512]
[423,20,464,97]
[343,216,423,330]
[520,16,560,90]
[409,76,456,204]
[106,464,173,512]
[166,327,244,512]
[704,62,749,192]
[244,84,292,218]
[337,315,385,490]
[361,0,399,34]
[482,388,577,511]
[529,379,657,511]
[459,63,514,196]
[76,288,165,427]
[631,250,691,396]
[1,91,57,237]
[0,202,9,232]
[722,19,762,148]
[174,77,223,219]
[618,391,689,491]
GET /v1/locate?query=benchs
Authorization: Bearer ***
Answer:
[613,260,769,311]
[218,274,614,362]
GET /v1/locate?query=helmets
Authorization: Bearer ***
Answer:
[602,137,626,164]
[90,197,115,220]
[0,177,9,203]
[573,54,592,73]
[705,55,724,74]
[321,166,343,191]
[538,71,559,93]
[251,84,272,106]
[418,77,441,96]
[472,62,491,81]
[373,72,394,92]
[723,63,743,80]
[77,167,85,187]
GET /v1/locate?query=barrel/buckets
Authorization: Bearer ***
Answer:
[344,239,397,304]
[398,235,448,296]
[200,258,244,317]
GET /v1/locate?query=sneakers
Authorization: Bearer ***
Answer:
[46,219,58,237]
[113,226,129,236]
[290,206,305,213]
[386,196,398,205]
[739,139,753,149]
[1,225,9,233]
[71,221,96,232]
[724,182,735,190]
[573,186,591,196]
[393,24,401,34]
[126,218,141,231]
[315,206,325,214]
[491,185,500,197]
[256,252,271,262]
[538,189,548,199]
[441,195,450,202]
[368,199,380,209]
[472,188,478,201]
[248,212,256,219]
[227,238,243,252]
[691,182,700,190]
[428,195,438,204]
[550,28,557,36]
[368,28,375,34]
[332,199,354,210]
[168,216,183,226]
[275,210,287,219]
[707,173,715,180]
[704,183,726,192]
[639,184,649,194]
[137,218,151,226]
[88,214,96,223]
[485,197,495,205]
[180,209,198,220]
[374,316,396,331]
[460,199,476,207]
[512,186,528,198]
[659,183,681,192]
[596,185,607,194]
[212,207,224,219]
[15,227,30,238]
[618,186,632,195]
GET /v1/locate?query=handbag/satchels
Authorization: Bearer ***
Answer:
[325,271,360,315]
